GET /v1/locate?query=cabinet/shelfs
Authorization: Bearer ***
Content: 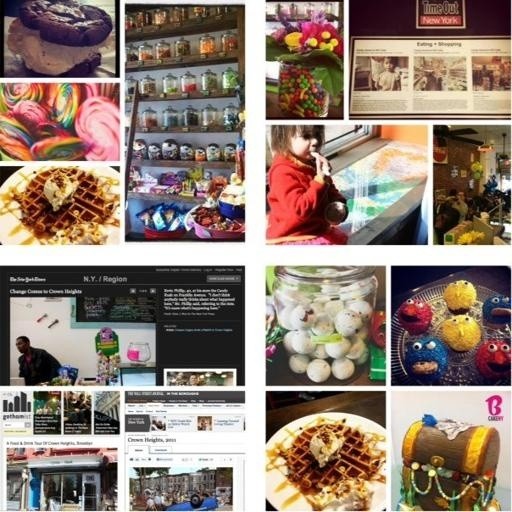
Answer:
[125,4,245,241]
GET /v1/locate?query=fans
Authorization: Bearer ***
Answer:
[434,125,484,147]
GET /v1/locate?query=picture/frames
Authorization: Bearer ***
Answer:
[416,0,467,30]
[349,35,511,119]
[163,368,237,386]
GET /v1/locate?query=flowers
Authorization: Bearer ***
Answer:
[266,5,344,95]
[456,230,486,244]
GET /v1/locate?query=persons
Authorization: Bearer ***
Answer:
[438,191,469,244]
[371,57,403,92]
[14,334,63,385]
[186,371,199,386]
[265,125,350,246]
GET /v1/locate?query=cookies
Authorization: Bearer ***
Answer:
[19,52,101,78]
[18,0,112,46]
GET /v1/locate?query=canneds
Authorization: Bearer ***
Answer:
[124,4,240,163]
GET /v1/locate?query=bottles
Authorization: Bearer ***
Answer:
[277,61,329,119]
[273,265,379,385]
[127,341,152,366]
[125,5,240,128]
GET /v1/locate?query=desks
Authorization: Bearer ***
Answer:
[118,362,156,386]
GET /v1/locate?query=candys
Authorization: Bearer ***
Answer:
[0,82,120,161]
[273,266,379,385]
[278,61,329,118]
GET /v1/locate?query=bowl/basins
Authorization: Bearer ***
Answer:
[324,202,348,225]
[187,209,245,239]
[391,282,511,386]
[140,220,187,239]
[217,200,245,219]
